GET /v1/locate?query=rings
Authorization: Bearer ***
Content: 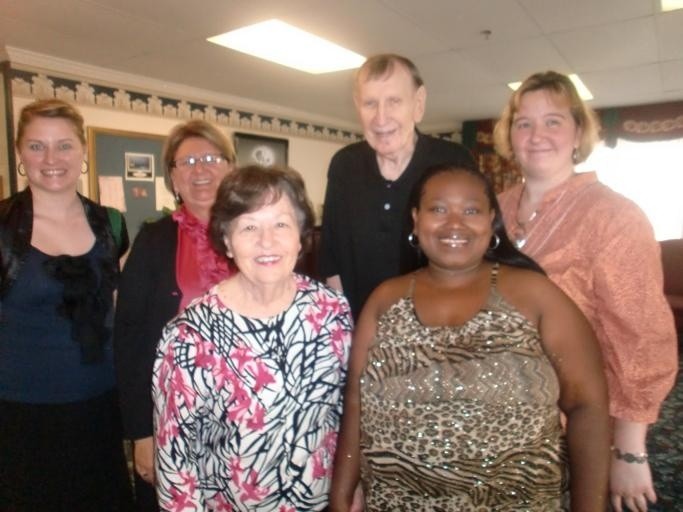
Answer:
[143,472,149,477]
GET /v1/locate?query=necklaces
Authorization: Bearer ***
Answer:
[513,178,565,248]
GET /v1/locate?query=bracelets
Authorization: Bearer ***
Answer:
[611,447,648,464]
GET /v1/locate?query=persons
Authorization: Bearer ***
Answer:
[150,164,366,511]
[1,102,131,511]
[487,67,678,512]
[109,115,244,510]
[320,53,503,320]
[325,162,611,511]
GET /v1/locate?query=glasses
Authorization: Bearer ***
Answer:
[172,151,224,166]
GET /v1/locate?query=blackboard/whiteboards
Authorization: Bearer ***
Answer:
[86,127,176,250]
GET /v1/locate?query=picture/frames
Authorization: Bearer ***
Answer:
[124,151,156,182]
